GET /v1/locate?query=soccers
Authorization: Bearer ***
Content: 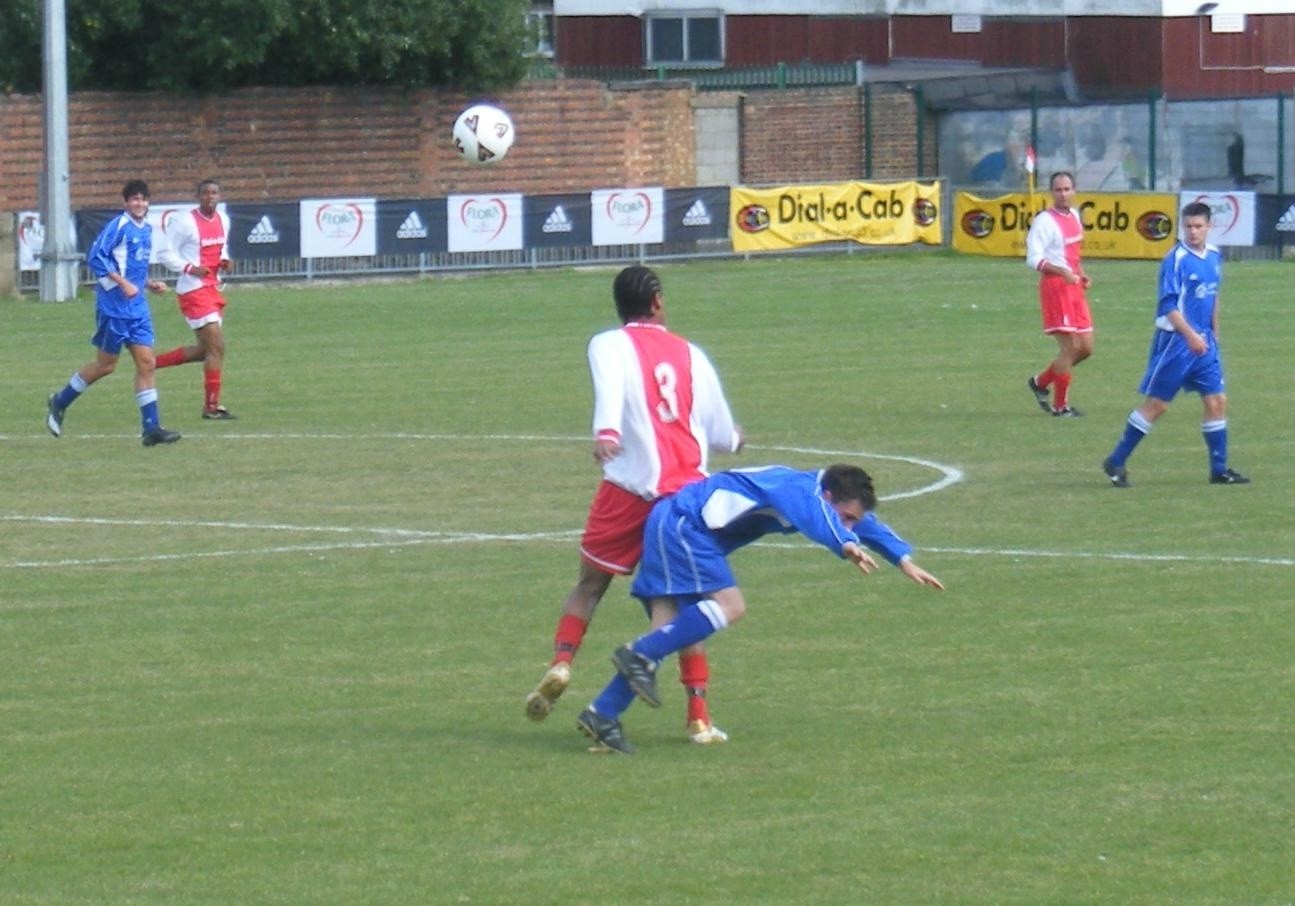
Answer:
[451,105,516,166]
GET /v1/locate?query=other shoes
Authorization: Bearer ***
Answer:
[687,719,728,745]
[524,661,571,721]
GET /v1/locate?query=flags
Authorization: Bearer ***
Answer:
[1024,146,1038,172]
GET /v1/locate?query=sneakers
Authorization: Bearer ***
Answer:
[47,393,64,437]
[1209,468,1250,484]
[1049,406,1087,417]
[202,406,236,420]
[611,639,660,709]
[1028,375,1051,412]
[142,428,181,448]
[1104,457,1129,488]
[575,704,639,755]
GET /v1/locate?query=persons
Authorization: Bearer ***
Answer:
[1028,173,1097,418]
[578,464,946,756]
[43,183,181,448]
[524,266,745,743]
[1100,203,1251,487]
[151,182,237,420]
